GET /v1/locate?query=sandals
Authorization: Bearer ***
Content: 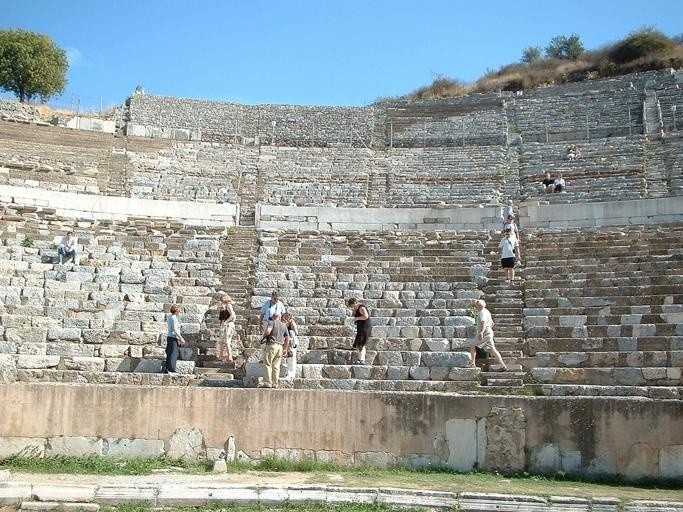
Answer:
[351,359,366,366]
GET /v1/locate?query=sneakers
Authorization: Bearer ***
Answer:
[463,362,516,373]
[256,385,277,389]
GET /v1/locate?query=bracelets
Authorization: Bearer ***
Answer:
[355,317,358,320]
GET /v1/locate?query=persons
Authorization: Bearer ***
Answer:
[553,173,565,193]
[280,312,299,379]
[259,289,286,364]
[541,172,555,193]
[502,215,518,255]
[469,299,509,372]
[216,293,236,363]
[256,311,289,387]
[497,228,520,287]
[572,146,581,159]
[347,298,371,365]
[51,231,76,264]
[567,144,575,161]
[165,304,185,372]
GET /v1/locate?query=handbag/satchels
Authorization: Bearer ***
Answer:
[282,351,293,358]
